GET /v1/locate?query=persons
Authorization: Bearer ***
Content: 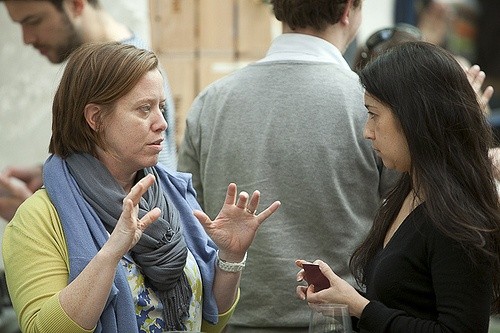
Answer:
[292,41,500,333]
[0,41,284,333]
[0,0,183,227]
[173,1,500,333]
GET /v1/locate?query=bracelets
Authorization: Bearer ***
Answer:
[213,248,248,273]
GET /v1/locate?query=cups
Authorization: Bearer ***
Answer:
[309,303,353,333]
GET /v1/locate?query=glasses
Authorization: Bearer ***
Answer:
[366,22,422,57]
